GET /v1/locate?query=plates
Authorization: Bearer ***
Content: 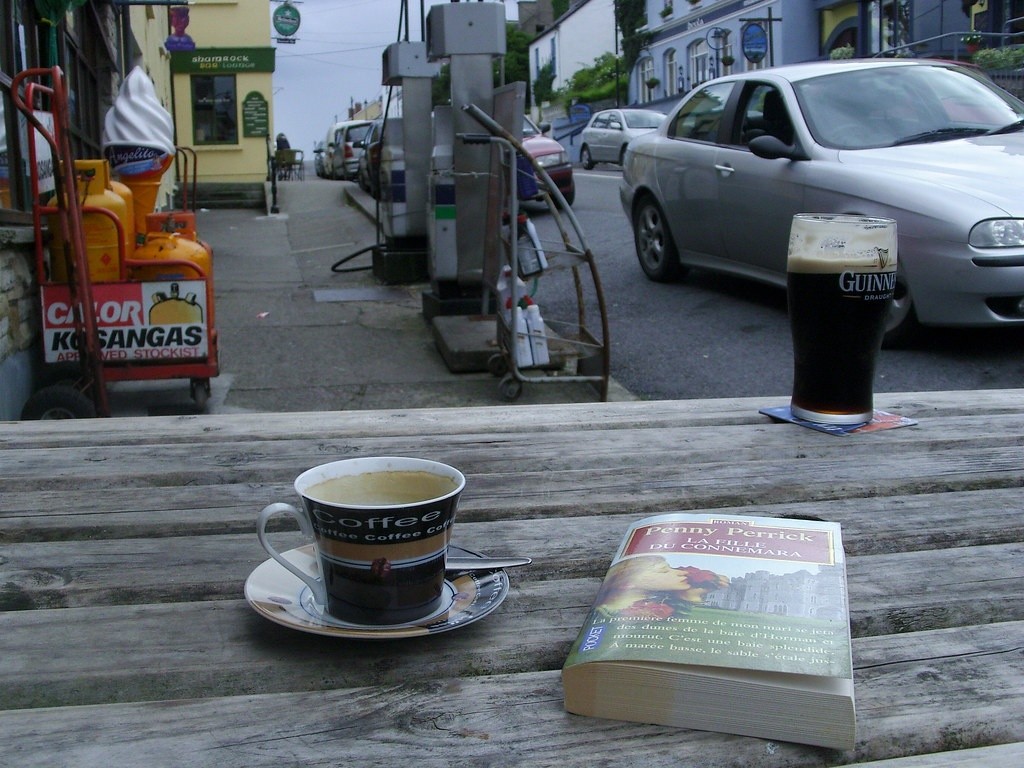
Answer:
[244,543,510,639]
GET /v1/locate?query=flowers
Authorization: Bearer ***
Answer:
[960,29,984,46]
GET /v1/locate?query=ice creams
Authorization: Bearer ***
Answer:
[102,65,175,235]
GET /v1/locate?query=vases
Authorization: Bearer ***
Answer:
[968,44,978,53]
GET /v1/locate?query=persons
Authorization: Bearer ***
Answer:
[273,133,291,180]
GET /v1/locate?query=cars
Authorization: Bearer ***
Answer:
[352,116,456,202]
[312,120,373,181]
[510,110,578,219]
[617,57,1024,352]
[578,107,669,170]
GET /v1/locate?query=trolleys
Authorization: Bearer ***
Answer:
[9,65,220,419]
[459,99,612,406]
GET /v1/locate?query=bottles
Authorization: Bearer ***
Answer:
[503,210,547,276]
[497,265,529,328]
[511,305,549,367]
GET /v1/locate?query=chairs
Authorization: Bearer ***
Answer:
[276,149,306,182]
[761,89,793,147]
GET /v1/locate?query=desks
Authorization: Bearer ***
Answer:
[0,392,1024,768]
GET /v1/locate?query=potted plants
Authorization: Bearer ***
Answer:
[721,55,735,67]
[645,78,660,89]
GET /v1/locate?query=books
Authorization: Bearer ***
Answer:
[564,512,857,751]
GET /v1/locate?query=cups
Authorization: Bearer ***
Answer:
[786,212,898,424]
[256,455,467,624]
